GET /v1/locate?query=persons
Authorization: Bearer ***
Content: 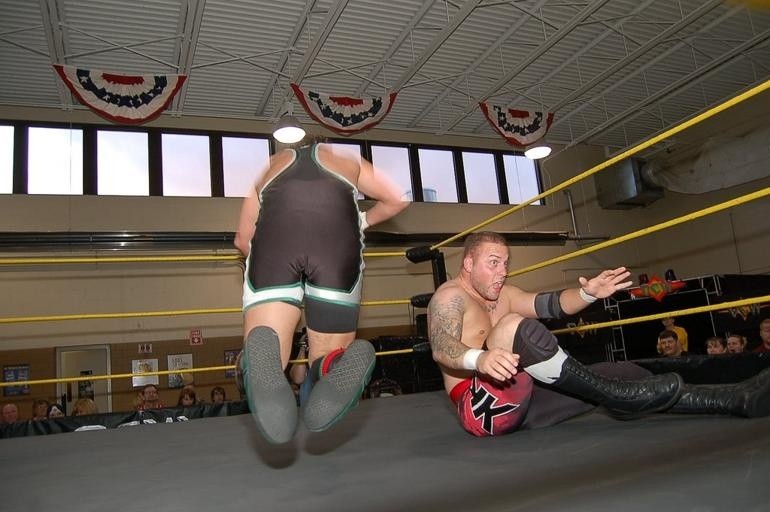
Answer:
[425,230,769,439]
[178,386,198,406]
[136,384,161,410]
[211,386,225,402]
[234,133,413,444]
[755,319,769,353]
[31,399,66,421]
[567,320,606,365]
[288,327,310,385]
[2,400,19,425]
[72,399,97,416]
[657,316,690,356]
[704,335,747,355]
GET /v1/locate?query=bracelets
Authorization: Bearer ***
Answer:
[360,212,368,230]
[580,288,598,304]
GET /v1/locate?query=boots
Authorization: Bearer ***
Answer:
[234,326,299,445]
[667,366,770,419]
[300,338,376,433]
[548,349,684,422]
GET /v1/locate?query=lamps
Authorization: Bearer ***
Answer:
[271,50,309,146]
[523,136,552,160]
